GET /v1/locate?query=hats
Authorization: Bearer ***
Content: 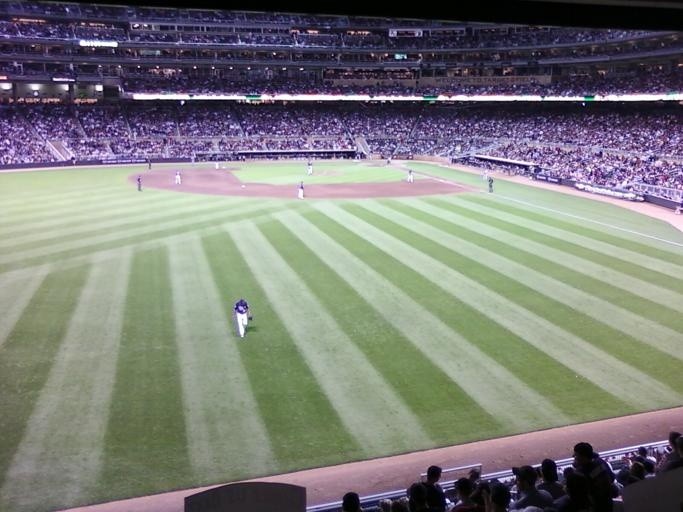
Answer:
[511,464,537,481]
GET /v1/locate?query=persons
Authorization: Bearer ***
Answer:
[234,299,250,337]
